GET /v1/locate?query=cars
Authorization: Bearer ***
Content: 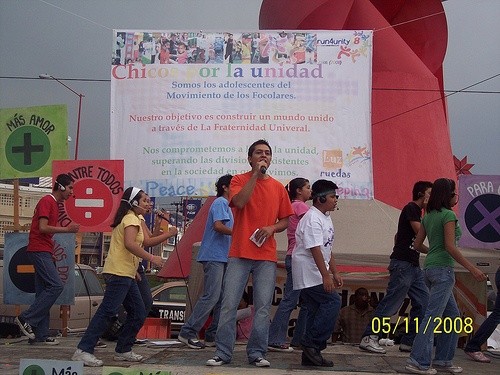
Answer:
[148,279,190,339]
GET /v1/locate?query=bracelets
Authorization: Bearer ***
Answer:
[150,255,153,262]
[271,225,276,232]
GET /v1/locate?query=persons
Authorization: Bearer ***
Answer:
[110,32,317,64]
[267,178,313,352]
[16,174,81,345]
[292,180,344,367]
[72,186,179,367]
[206,138,294,369]
[177,174,243,349]
[364,181,500,375]
[337,287,378,344]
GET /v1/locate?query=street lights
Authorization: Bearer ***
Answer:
[37,72,86,160]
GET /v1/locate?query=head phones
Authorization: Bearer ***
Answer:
[445,177,455,198]
[417,181,425,198]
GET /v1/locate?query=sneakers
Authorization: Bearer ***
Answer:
[133,341,147,346]
[379,338,393,345]
[71,349,104,367]
[465,350,491,363]
[268,344,294,352]
[16,316,35,338]
[178,333,202,348]
[359,334,387,353]
[399,343,412,352]
[405,363,437,375]
[302,348,335,366]
[114,352,143,361]
[253,357,271,366]
[205,337,216,347]
[432,364,463,373]
[31,337,59,345]
[94,341,107,348]
[206,356,226,365]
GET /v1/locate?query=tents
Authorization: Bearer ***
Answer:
[272,197,500,350]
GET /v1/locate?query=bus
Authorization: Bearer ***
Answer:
[0,257,107,338]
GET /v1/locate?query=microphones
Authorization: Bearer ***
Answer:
[311,191,326,203]
[134,204,150,213]
[55,180,66,191]
[455,194,459,205]
[260,160,266,173]
[153,209,170,223]
[128,187,137,206]
